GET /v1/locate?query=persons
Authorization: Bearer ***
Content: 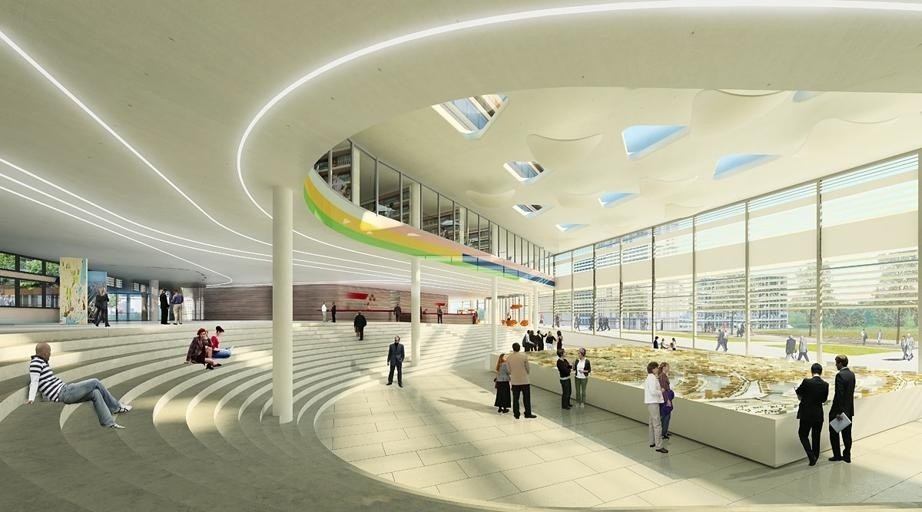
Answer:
[353,311,366,340]
[207,325,231,358]
[571,347,591,408]
[657,362,673,440]
[876,327,883,344]
[495,353,511,413]
[93,286,110,327]
[159,289,170,324]
[472,311,479,324]
[331,302,336,322]
[538,313,547,329]
[795,362,829,466]
[506,311,511,320]
[573,312,582,331]
[643,362,668,453]
[588,315,610,331]
[170,289,183,325]
[25,342,133,429]
[900,333,915,360]
[860,328,867,346]
[422,308,429,313]
[552,314,560,328]
[715,329,728,352]
[737,322,745,337]
[185,328,222,369]
[386,336,404,387]
[556,349,573,410]
[393,303,401,322]
[436,305,443,323]
[653,336,676,351]
[785,334,809,362]
[321,302,329,322]
[522,329,563,352]
[829,355,855,463]
[505,343,537,419]
